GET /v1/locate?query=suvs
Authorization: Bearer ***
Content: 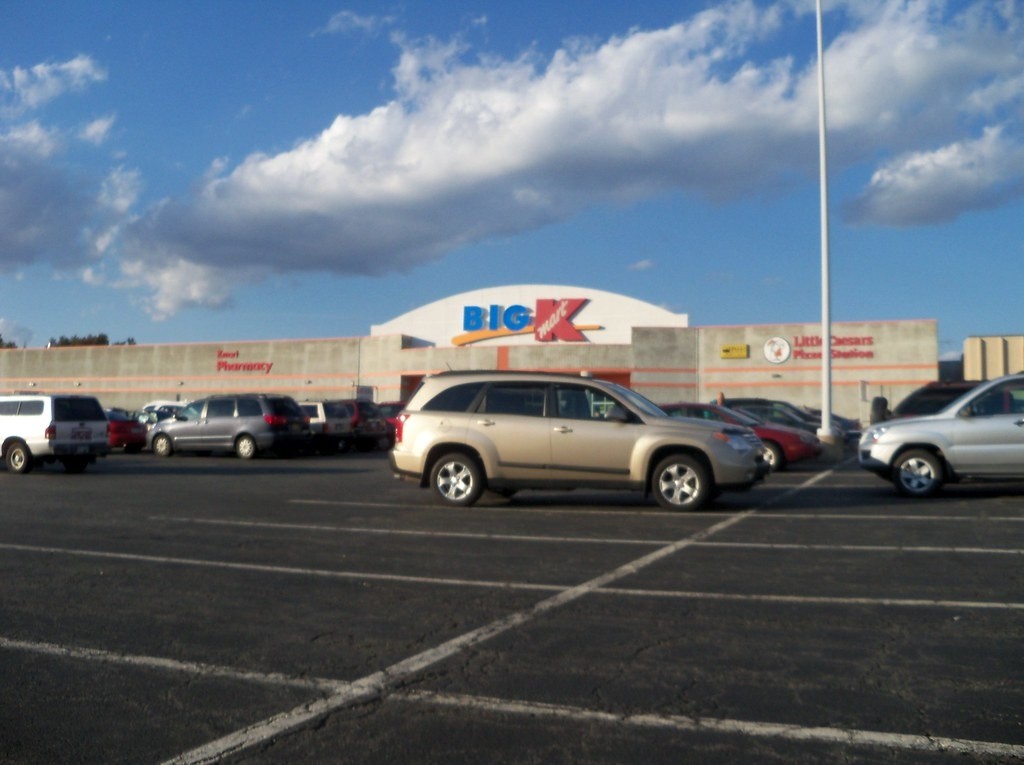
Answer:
[389,369,770,511]
[0,392,108,475]
[376,401,407,426]
[146,392,312,459]
[870,381,1024,427]
[344,400,386,452]
[298,401,352,455]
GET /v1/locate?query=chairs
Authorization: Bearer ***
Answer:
[560,390,591,419]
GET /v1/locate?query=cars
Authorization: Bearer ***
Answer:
[139,399,192,425]
[658,402,822,471]
[714,395,852,443]
[104,408,146,452]
[856,371,1024,496]
